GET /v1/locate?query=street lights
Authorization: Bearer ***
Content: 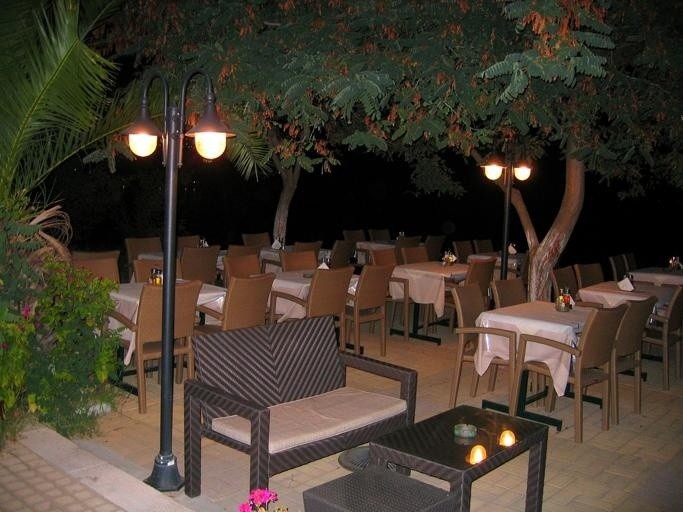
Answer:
[122,65,242,495]
[479,129,533,279]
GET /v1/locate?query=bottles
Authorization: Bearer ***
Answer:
[275,236,286,250]
[149,268,161,285]
[443,250,453,261]
[559,286,570,304]
[623,271,634,280]
[321,257,331,266]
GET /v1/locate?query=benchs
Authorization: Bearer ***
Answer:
[181,315,417,500]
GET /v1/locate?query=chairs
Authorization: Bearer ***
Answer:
[450,251,682,444]
[70,234,278,414]
[262,228,438,357]
